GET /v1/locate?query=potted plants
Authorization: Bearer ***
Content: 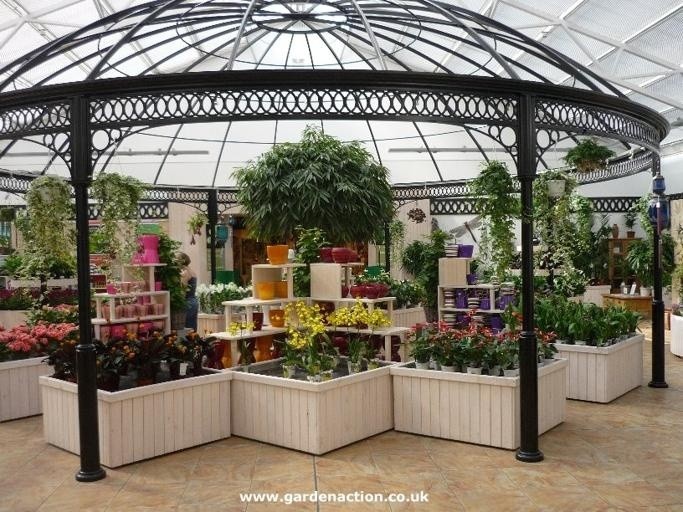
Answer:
[534,290,646,348]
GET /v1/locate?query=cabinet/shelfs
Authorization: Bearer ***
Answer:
[91,262,171,344]
[437,282,507,339]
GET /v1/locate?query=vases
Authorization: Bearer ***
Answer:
[441,363,519,377]
[252,244,388,301]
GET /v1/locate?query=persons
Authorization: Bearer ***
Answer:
[171,251,198,333]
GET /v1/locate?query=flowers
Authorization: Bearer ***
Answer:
[226,296,393,380]
[407,309,559,369]
[194,281,251,314]
[0,318,224,385]
